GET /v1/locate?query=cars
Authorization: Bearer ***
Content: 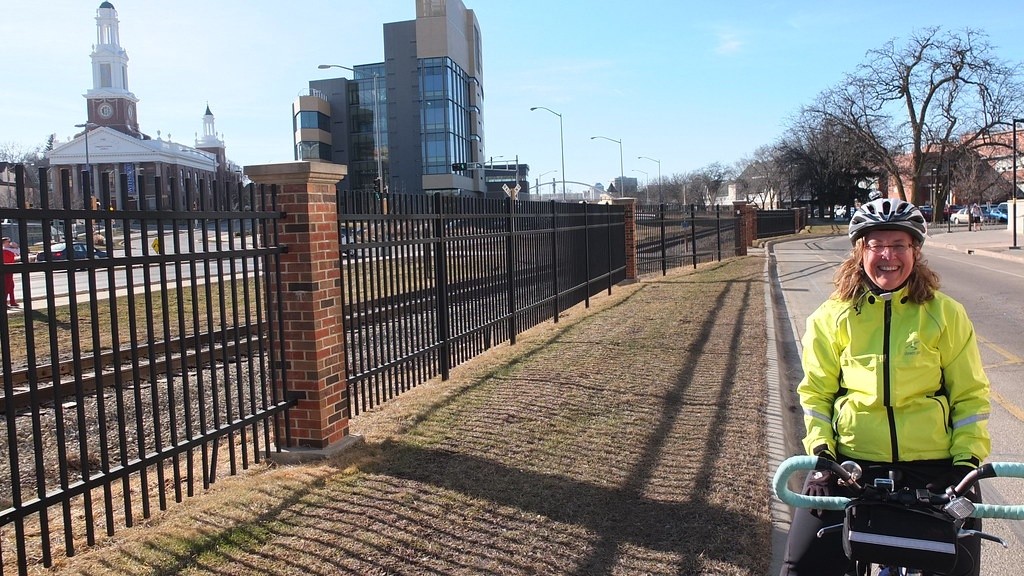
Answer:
[814,205,855,218]
[943,202,1008,222]
[446,219,466,228]
[37,241,107,272]
[918,206,933,222]
[950,207,988,225]
[340,226,359,257]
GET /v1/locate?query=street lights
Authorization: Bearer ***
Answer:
[317,64,383,196]
[1008,118,1024,249]
[632,169,649,205]
[74,123,89,171]
[530,107,565,200]
[638,156,662,204]
[539,169,557,199]
[590,136,623,197]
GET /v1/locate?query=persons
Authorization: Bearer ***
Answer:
[779,197,990,576]
[970,202,983,231]
[2,237,19,308]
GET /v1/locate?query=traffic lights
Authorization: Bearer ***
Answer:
[452,163,467,171]
[373,177,383,194]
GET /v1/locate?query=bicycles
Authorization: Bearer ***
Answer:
[772,455,1024,576]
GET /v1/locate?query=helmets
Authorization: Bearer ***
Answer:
[848,197,928,243]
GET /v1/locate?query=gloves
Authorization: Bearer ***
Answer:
[942,465,976,502]
[800,453,842,517]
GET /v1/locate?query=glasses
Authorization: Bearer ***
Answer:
[863,240,916,253]
